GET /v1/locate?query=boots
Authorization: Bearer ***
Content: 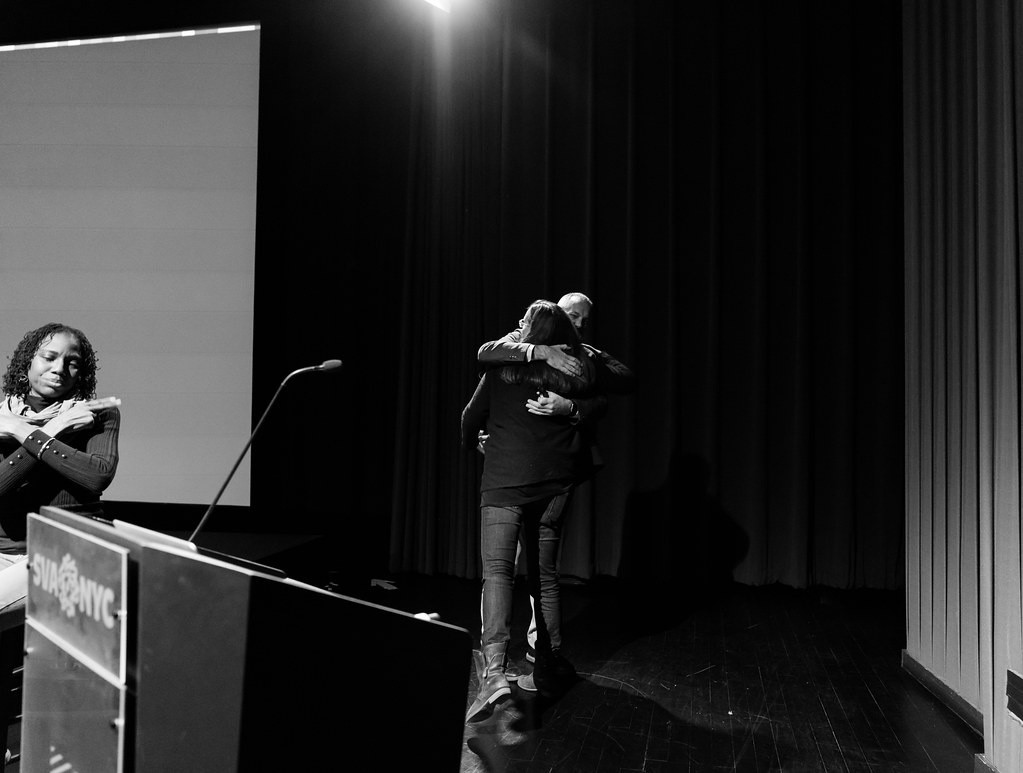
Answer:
[463,640,512,725]
[516,641,557,693]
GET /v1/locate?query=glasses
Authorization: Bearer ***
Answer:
[518,318,531,328]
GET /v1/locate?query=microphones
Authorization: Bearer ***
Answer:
[187,359,345,543]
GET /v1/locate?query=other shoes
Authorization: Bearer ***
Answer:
[524,647,537,664]
[490,650,522,683]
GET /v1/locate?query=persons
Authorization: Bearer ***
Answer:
[458,299,594,725]
[476,292,631,693]
[0,321,124,560]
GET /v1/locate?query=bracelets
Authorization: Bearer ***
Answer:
[37,436,56,459]
[569,400,581,421]
